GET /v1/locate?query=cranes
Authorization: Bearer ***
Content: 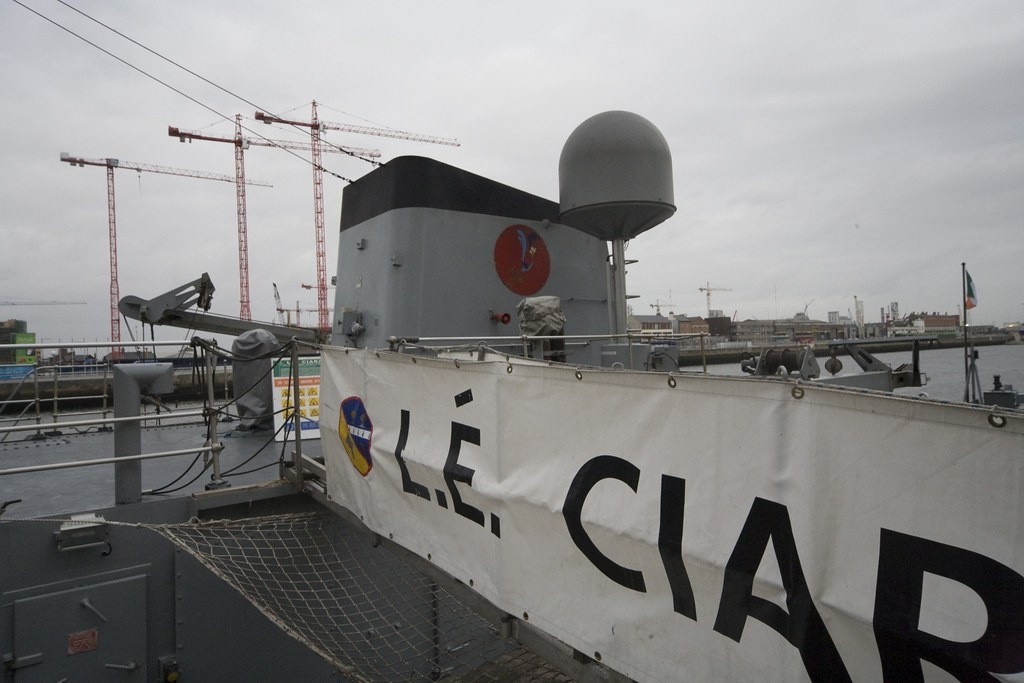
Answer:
[269,280,336,328]
[0,292,89,306]
[252,98,462,332]
[60,151,275,363]
[164,112,383,322]
[650,298,677,315]
[699,282,732,318]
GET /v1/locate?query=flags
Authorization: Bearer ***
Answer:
[964,268,977,309]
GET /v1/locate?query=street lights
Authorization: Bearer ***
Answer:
[41,337,51,360]
